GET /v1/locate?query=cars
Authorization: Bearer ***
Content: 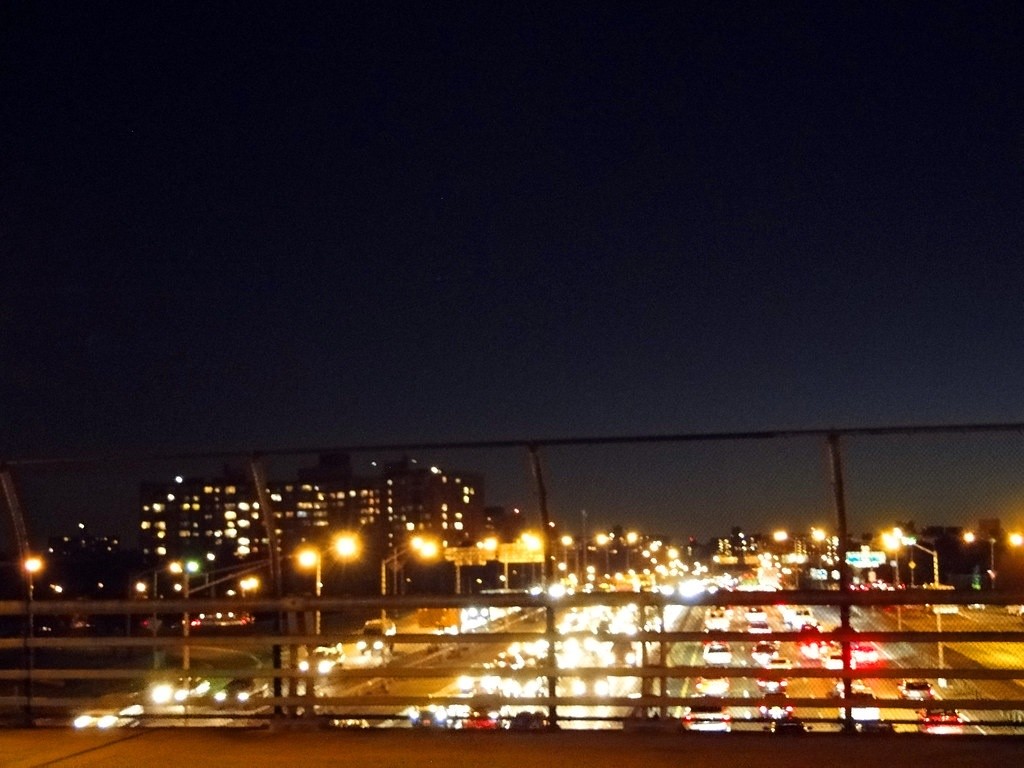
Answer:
[72,580,968,736]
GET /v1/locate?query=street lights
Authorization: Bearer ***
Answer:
[376,527,647,653]
[964,531,995,590]
[316,534,361,634]
[878,531,904,635]
[180,546,319,673]
[890,532,943,677]
